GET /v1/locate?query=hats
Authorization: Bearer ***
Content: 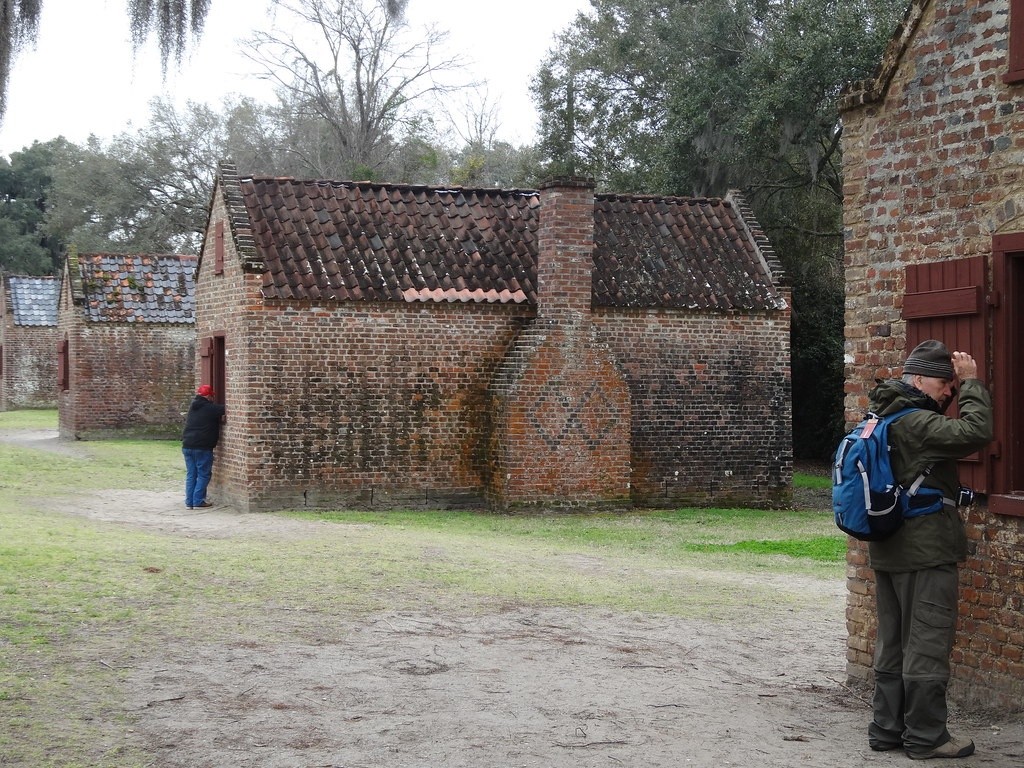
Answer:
[198,385,217,397]
[900,339,954,378]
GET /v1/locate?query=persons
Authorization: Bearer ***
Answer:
[865,337,995,761]
[181,384,225,510]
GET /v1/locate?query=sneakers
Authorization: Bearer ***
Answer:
[193,501,213,509]
[185,503,193,510]
[906,737,975,760]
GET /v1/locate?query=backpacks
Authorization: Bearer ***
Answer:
[831,409,955,542]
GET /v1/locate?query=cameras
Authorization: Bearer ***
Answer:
[958,487,988,507]
